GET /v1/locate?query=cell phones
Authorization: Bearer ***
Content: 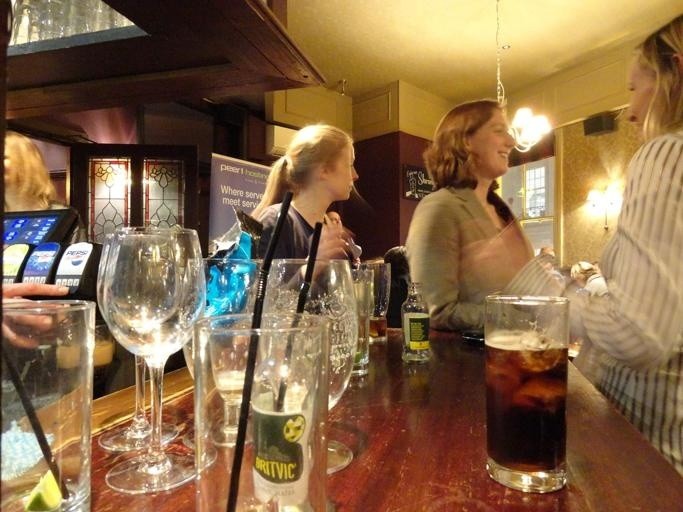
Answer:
[462,330,484,346]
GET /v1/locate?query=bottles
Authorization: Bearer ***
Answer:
[400,282,433,365]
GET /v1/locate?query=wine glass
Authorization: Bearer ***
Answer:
[257,260,357,473]
[178,258,274,448]
[96,235,181,450]
[105,228,207,494]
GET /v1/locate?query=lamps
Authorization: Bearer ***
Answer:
[493,0,554,154]
[581,181,624,233]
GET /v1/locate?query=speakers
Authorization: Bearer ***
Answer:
[583,111,615,136]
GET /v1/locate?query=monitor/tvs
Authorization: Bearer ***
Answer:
[2,208,80,246]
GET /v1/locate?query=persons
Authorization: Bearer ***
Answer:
[373,246,412,328]
[1,283,69,350]
[1,130,85,247]
[501,15,683,477]
[251,124,359,315]
[327,212,362,272]
[406,99,546,334]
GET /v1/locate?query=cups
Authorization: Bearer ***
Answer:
[194,312,330,512]
[0,301,95,512]
[350,270,373,377]
[356,263,391,346]
[201,314,251,447]
[485,296,570,492]
